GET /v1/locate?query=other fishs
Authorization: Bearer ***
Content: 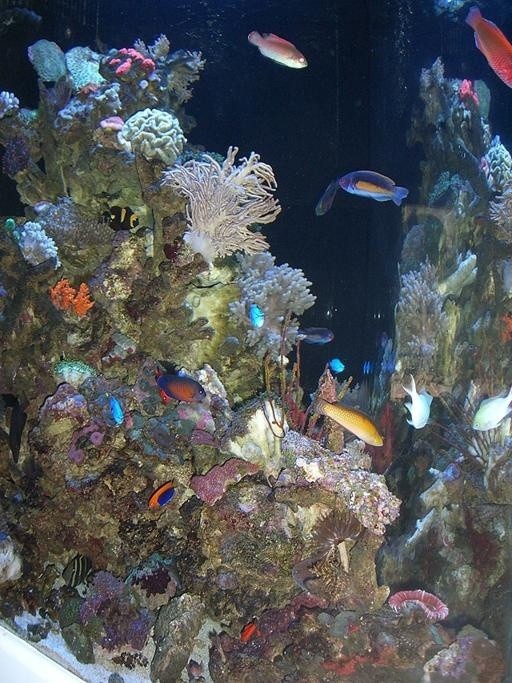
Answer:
[0,7,512,643]
